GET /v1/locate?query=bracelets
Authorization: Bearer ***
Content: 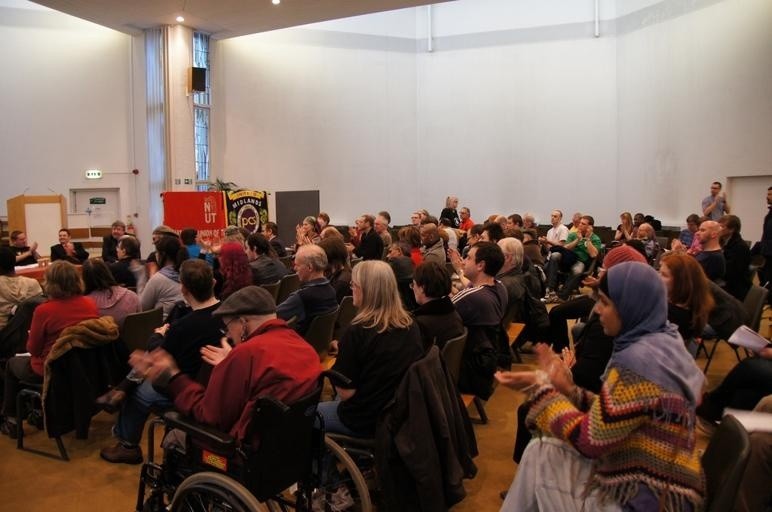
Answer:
[518,368,550,394]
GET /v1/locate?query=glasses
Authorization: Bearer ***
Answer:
[218,317,237,335]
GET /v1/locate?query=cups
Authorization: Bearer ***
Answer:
[37,257,50,270]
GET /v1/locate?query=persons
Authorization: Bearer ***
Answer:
[757,185,772,305]
[701,180,733,222]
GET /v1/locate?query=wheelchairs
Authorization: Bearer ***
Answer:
[136,370,373,511]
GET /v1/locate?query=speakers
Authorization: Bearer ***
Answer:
[192,67,206,91]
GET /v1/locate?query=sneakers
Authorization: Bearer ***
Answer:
[0,420,25,440]
[695,414,719,435]
[311,484,355,512]
[100,442,144,465]
[95,388,127,415]
[539,289,569,304]
[27,410,44,431]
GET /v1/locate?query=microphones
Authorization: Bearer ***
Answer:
[47,187,56,192]
[24,187,29,193]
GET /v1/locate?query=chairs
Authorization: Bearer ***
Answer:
[0,221,772,512]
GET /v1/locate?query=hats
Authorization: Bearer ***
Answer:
[211,285,277,318]
[153,225,180,239]
[602,244,647,269]
